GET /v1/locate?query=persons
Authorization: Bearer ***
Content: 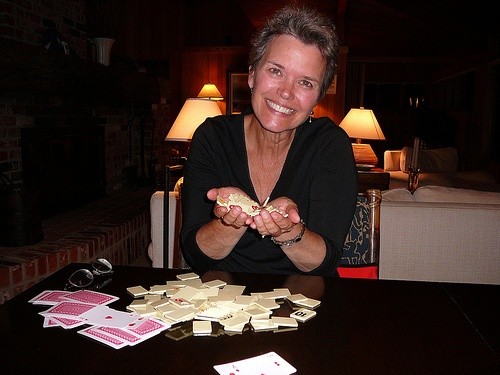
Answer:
[179,5,359,279]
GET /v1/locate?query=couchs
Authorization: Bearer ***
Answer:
[377,144,500,286]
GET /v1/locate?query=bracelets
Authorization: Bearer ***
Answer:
[271,219,306,246]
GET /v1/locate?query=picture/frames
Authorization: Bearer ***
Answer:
[225,70,253,116]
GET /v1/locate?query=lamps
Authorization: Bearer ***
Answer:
[198,83,222,98]
[164,98,222,142]
[338,108,386,166]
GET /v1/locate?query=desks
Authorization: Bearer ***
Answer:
[0,262,500,375]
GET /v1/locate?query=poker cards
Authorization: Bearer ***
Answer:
[27,286,169,349]
[213,351,297,375]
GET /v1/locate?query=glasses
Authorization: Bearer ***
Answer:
[63,258,112,291]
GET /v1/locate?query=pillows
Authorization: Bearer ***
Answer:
[400,146,458,173]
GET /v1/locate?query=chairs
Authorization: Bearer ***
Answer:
[173,176,382,279]
